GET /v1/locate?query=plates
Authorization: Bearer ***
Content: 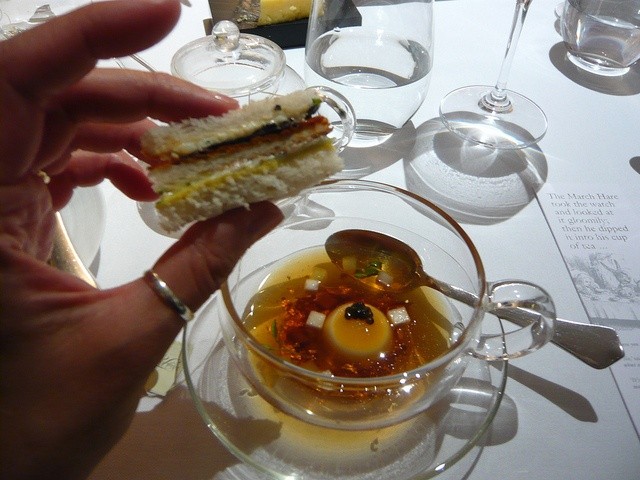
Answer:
[181,295,522,480]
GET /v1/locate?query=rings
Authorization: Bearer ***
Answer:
[143,270,194,321]
[31,169,50,184]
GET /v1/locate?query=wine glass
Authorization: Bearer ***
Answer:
[439,0,550,151]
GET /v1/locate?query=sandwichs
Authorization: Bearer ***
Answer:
[137,86,343,232]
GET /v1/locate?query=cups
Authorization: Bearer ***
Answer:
[559,1,640,77]
[302,0,436,149]
[59,186,105,282]
[218,178,558,433]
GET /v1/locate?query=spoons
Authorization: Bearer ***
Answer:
[325,228,625,370]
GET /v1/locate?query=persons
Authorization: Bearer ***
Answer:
[1,0,285,479]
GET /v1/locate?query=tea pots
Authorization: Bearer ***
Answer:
[110,19,357,155]
